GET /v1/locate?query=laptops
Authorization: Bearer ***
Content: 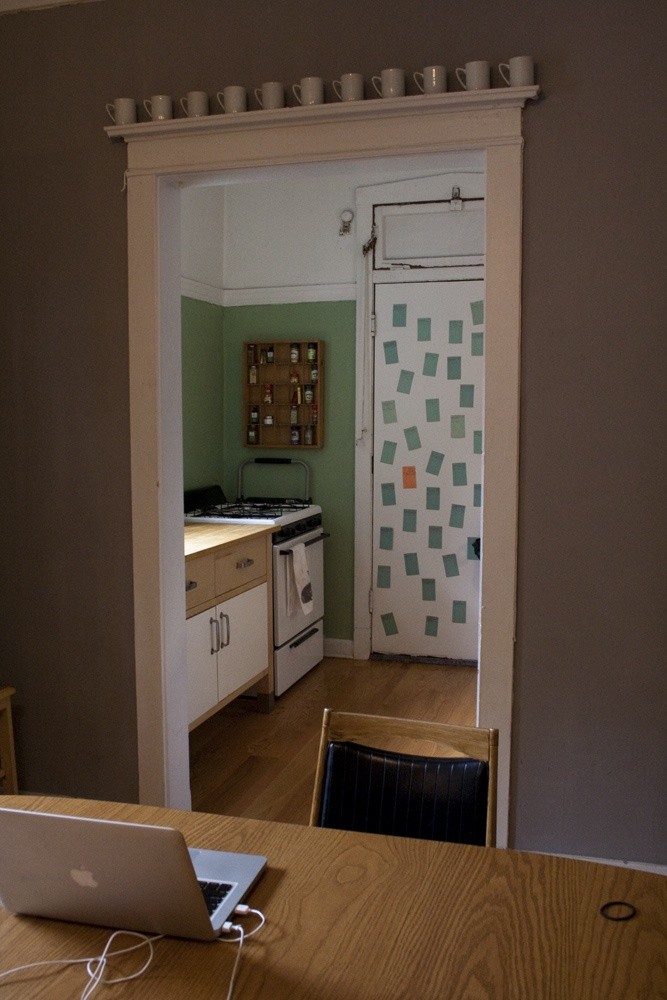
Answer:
[1,807,267,940]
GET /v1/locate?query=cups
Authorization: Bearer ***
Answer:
[413,66,448,94]
[180,91,209,118]
[456,61,491,91]
[216,86,246,114]
[331,72,364,102]
[292,76,324,106]
[497,56,534,88]
[106,98,136,125]
[254,81,284,110]
[371,69,405,98]
[143,95,173,121]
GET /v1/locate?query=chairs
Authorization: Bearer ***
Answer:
[307,706,500,850]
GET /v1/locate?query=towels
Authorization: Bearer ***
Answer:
[285,542,315,617]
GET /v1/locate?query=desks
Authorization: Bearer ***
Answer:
[0,792,667,1000]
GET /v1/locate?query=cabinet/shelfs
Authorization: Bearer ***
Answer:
[0,684,20,795]
[240,338,327,449]
[185,520,281,733]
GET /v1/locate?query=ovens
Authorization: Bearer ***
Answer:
[273,527,331,697]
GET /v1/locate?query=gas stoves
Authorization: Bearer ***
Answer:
[183,484,322,545]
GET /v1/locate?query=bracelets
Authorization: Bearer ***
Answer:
[599,901,638,922]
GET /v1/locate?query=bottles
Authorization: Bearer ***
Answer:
[249,366,258,384]
[267,347,273,362]
[307,344,317,363]
[310,364,318,383]
[304,385,314,404]
[312,405,317,424]
[290,406,298,424]
[263,415,274,424]
[263,388,272,404]
[247,345,256,364]
[250,407,259,423]
[290,344,301,362]
[304,426,313,444]
[248,432,257,444]
[290,426,300,444]
